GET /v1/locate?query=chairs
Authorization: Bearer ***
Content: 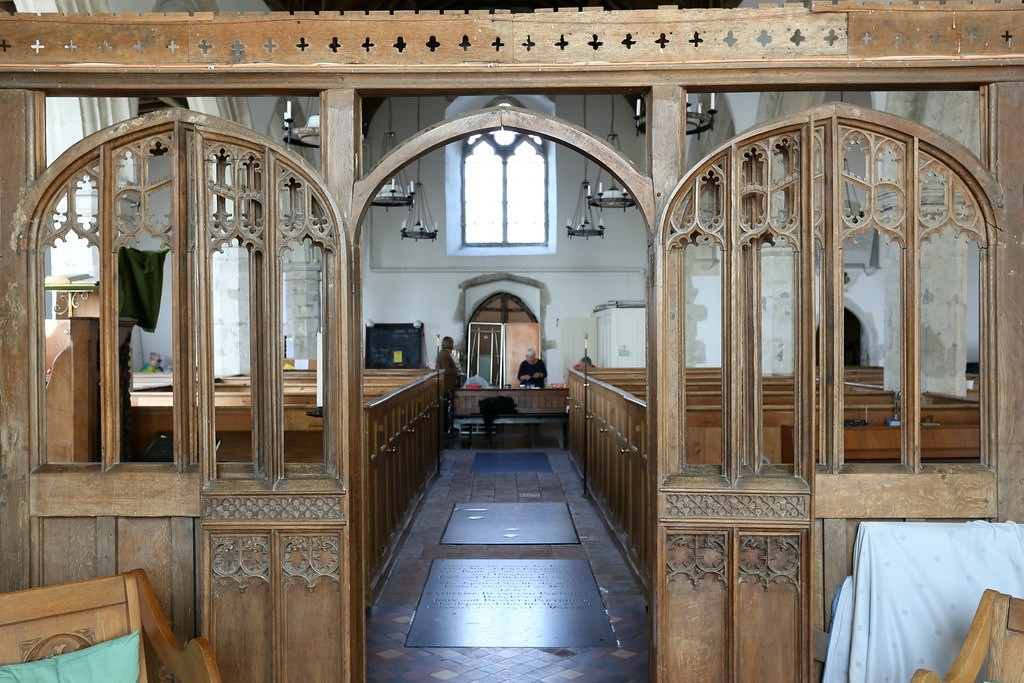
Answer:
[908,589,1024,683]
[0,568,224,683]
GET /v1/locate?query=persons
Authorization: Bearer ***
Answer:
[437,336,467,448]
[517,348,547,433]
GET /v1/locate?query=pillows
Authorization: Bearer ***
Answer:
[0,630,140,683]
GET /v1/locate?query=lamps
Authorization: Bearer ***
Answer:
[565,90,718,240]
[413,320,422,329]
[367,319,375,327]
[281,95,438,242]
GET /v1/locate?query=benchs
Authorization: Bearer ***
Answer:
[130,366,438,467]
[566,367,980,605]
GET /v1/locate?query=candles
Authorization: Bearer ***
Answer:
[317,332,324,408]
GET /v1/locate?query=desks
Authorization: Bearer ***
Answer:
[454,411,568,454]
